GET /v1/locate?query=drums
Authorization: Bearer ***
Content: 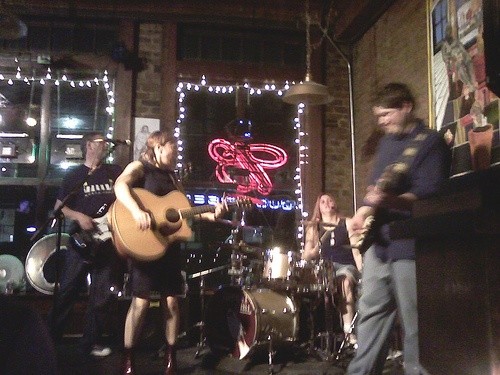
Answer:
[202,280,303,361]
[293,257,335,295]
[26,231,85,297]
[237,221,273,255]
[0,250,27,295]
[262,247,302,291]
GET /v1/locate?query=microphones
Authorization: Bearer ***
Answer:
[105,138,132,146]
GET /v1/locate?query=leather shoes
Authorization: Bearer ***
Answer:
[163,343,178,374]
[123,347,135,374]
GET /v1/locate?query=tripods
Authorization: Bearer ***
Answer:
[294,222,358,375]
[157,261,233,360]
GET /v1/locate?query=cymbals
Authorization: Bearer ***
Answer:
[299,219,336,230]
[230,190,268,207]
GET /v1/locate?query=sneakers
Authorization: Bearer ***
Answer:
[79,342,112,357]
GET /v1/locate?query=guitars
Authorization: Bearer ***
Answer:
[67,162,200,264]
[108,186,255,262]
[354,133,438,253]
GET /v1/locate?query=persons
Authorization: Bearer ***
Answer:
[304,192,363,350]
[49,131,124,356]
[113,132,229,375]
[347,82,451,375]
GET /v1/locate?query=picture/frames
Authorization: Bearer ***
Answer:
[426,0,500,182]
